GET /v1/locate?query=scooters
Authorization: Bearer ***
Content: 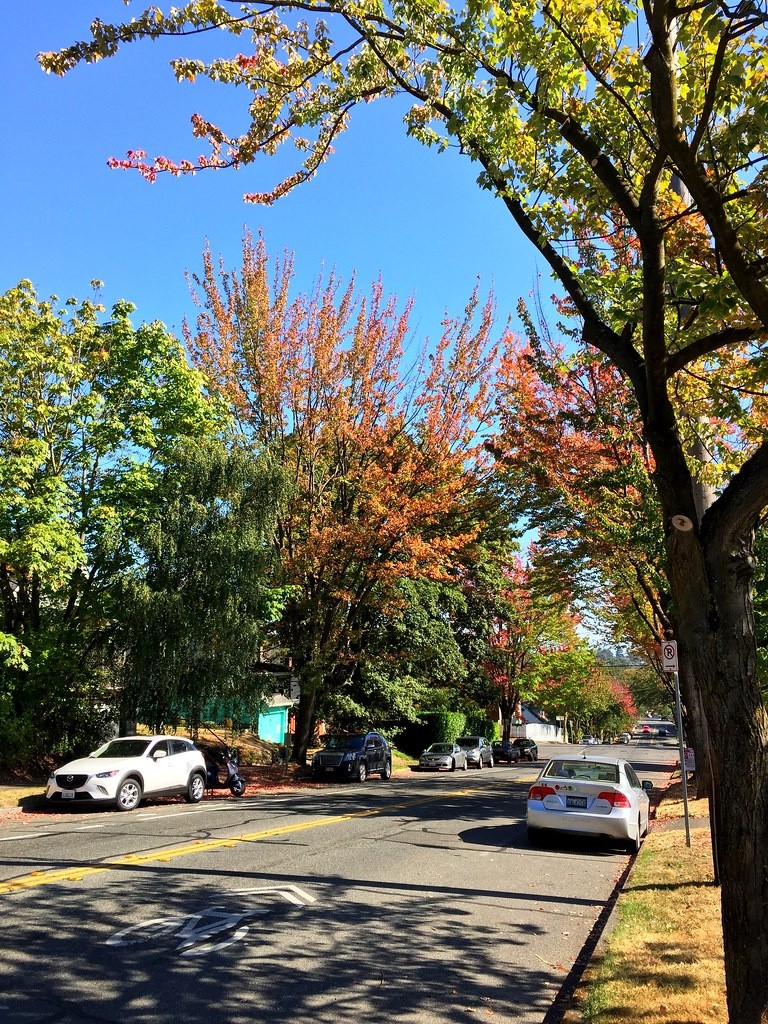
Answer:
[197,744,247,796]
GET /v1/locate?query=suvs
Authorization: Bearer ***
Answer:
[456,736,494,769]
[513,739,538,761]
[313,733,391,782]
[45,736,208,811]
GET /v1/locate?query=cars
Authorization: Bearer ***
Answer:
[579,730,631,745]
[492,740,520,763]
[643,726,651,732]
[419,743,467,772]
[527,755,654,854]
[659,725,678,737]
